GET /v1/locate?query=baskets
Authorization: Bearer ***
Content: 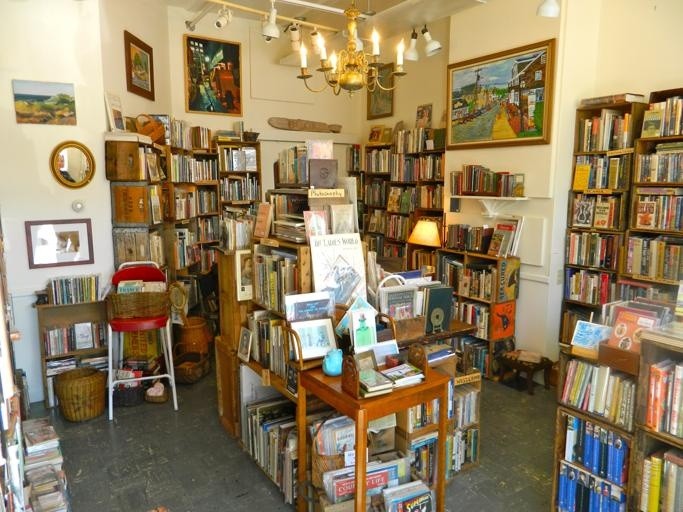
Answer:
[107,284,173,321]
[178,313,215,361]
[170,341,214,386]
[51,364,109,425]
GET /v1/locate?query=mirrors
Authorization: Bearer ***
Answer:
[48,139,97,190]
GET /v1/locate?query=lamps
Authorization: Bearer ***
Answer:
[405,25,421,62]
[261,1,280,46]
[536,0,563,20]
[295,0,409,99]
[420,24,443,57]
[208,1,327,48]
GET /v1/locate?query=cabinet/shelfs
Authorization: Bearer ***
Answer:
[105,108,261,406]
[551,88,683,511]
[31,293,111,411]
[216,138,482,510]
[0,215,71,512]
[348,118,527,379]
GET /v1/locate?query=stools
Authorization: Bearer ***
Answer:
[500,351,553,394]
[107,260,178,420]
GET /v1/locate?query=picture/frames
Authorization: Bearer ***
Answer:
[22,216,96,270]
[365,61,396,122]
[122,28,156,102]
[182,32,245,119]
[443,36,559,151]
